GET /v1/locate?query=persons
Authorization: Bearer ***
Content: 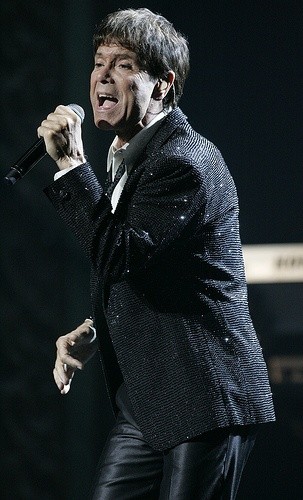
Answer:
[36,4,278,500]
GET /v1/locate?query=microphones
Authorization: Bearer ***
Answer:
[4,103,85,185]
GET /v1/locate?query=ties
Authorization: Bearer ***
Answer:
[102,159,127,210]
